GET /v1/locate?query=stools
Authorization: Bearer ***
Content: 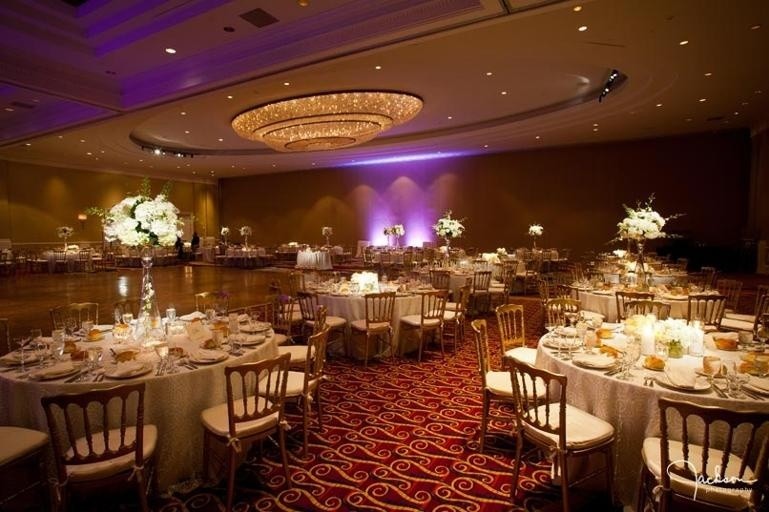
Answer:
[0,426,52,511]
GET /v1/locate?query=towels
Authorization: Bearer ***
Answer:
[108,357,153,378]
[34,358,83,379]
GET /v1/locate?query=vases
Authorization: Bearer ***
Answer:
[129,248,172,348]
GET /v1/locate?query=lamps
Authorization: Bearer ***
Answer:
[229,87,427,155]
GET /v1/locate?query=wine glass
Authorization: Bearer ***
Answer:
[544,256,769,401]
[2,292,273,383]
[292,264,436,294]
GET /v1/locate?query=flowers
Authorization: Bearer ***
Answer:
[81,175,192,250]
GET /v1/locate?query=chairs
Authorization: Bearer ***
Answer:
[0,226,197,276]
[48,300,100,334]
[193,175,764,511]
[38,380,164,512]
[110,295,150,326]
[0,316,13,354]
